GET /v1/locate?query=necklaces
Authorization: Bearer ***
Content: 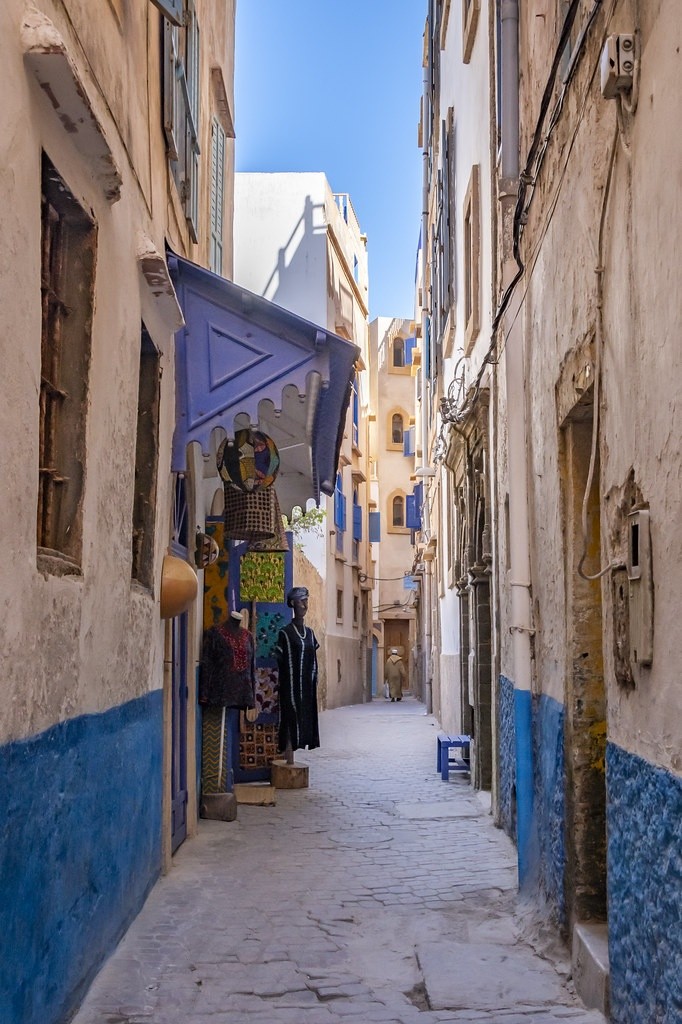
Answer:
[291,622,307,640]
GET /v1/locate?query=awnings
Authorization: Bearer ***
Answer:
[166,249,362,516]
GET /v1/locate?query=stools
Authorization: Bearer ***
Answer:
[437,735,470,782]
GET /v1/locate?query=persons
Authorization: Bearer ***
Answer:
[199,611,256,710]
[384,650,406,703]
[271,587,320,754]
